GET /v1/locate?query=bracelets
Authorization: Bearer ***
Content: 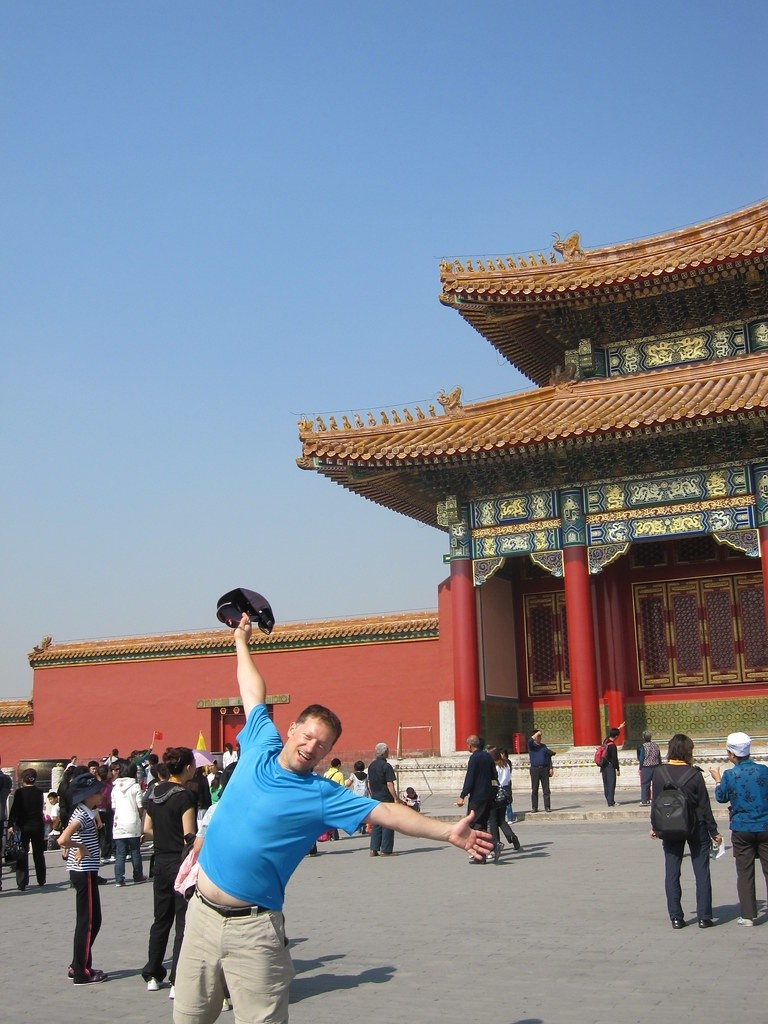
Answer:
[716,782,721,785]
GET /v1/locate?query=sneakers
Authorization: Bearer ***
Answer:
[16,852,148,891]
[68,965,107,985]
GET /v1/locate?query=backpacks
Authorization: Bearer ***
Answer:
[594,739,614,767]
[653,765,702,839]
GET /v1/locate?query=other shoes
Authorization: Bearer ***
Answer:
[610,802,619,806]
[639,800,650,805]
[221,997,229,1012]
[737,916,753,926]
[358,806,551,864]
[148,976,163,991]
[169,985,175,998]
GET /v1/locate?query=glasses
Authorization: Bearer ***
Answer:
[112,768,120,771]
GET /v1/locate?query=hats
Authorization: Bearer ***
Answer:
[726,732,751,757]
[70,773,104,804]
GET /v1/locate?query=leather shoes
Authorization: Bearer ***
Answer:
[699,918,714,928]
[672,919,686,929]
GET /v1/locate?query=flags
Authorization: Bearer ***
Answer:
[197,733,206,751]
[155,731,163,740]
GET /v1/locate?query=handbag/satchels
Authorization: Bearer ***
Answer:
[491,786,513,809]
[4,829,27,864]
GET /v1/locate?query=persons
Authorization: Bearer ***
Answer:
[709,732,768,926]
[172,612,494,1024]
[306,743,421,857]
[649,734,722,928]
[142,747,239,1012]
[600,722,625,806]
[527,730,553,813]
[457,735,520,863]
[638,732,662,806]
[0,731,240,892]
[57,773,108,986]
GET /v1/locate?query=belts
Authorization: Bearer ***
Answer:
[532,765,546,768]
[195,889,270,917]
